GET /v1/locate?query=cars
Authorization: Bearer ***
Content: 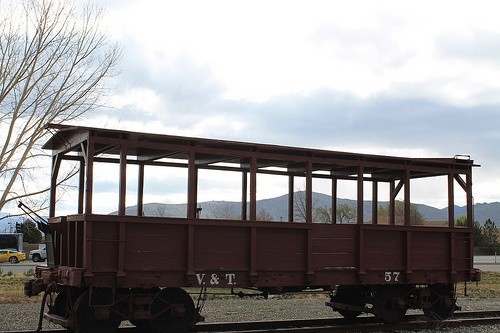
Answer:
[0,249,25,264]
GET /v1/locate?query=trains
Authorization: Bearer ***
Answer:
[15,121,482,332]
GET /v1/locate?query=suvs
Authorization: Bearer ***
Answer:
[29,246,47,262]
